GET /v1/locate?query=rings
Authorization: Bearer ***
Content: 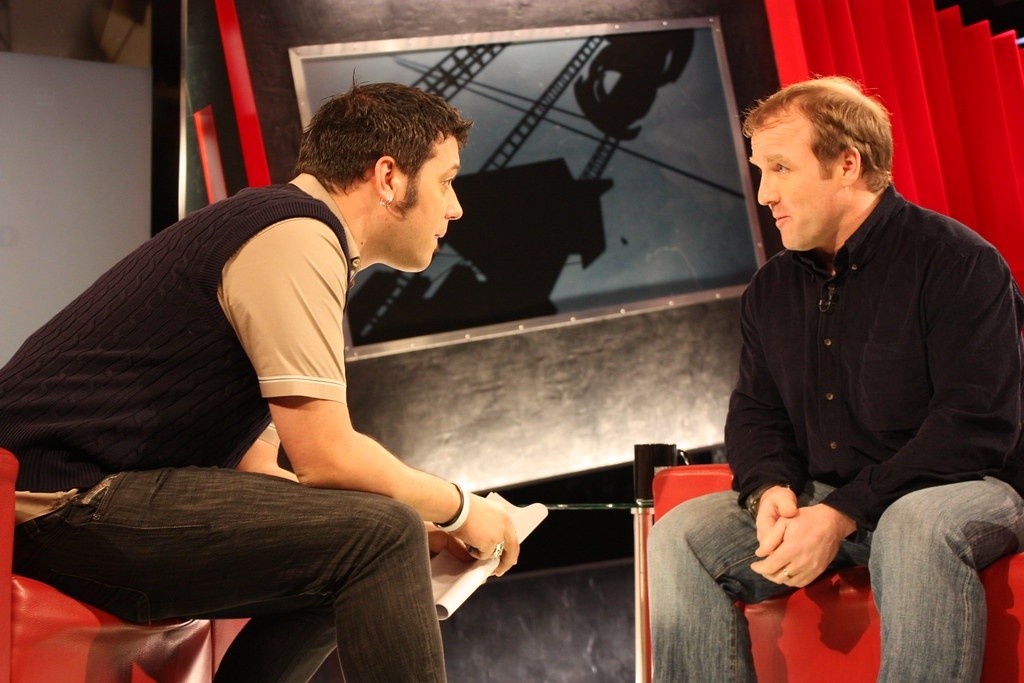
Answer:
[494,540,504,558]
[782,566,793,579]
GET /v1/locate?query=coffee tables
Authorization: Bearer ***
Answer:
[518,504,655,683]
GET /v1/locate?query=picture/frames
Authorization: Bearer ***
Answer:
[286,16,766,363]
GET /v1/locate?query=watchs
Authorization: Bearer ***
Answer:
[744,481,790,522]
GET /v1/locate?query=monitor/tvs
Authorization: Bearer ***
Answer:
[289,16,767,363]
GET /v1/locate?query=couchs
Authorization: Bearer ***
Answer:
[0,448,250,683]
[653,462,1024,683]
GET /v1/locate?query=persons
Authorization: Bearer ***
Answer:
[647,75,1024,683]
[0,66,519,683]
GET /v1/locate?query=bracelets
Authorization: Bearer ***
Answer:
[432,481,470,534]
